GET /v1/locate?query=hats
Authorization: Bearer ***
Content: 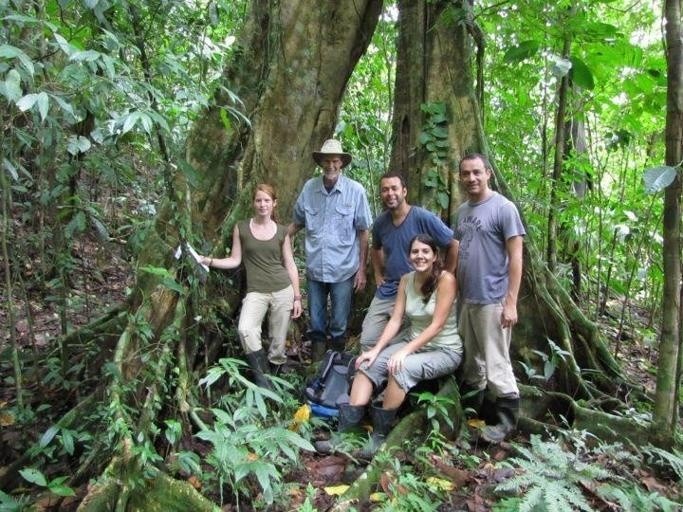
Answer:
[309,138,353,172]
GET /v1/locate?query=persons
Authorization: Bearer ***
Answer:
[283,138,372,370]
[196,183,302,396]
[456,152,523,446]
[356,174,460,363]
[312,231,466,465]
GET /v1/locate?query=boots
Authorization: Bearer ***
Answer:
[266,357,283,388]
[458,383,484,416]
[308,333,326,363]
[475,390,520,444]
[245,345,274,405]
[311,401,367,455]
[331,336,345,354]
[349,398,399,461]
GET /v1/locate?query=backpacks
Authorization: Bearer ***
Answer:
[303,349,358,418]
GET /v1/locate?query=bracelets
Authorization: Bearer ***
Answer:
[293,295,302,300]
[209,256,213,266]
